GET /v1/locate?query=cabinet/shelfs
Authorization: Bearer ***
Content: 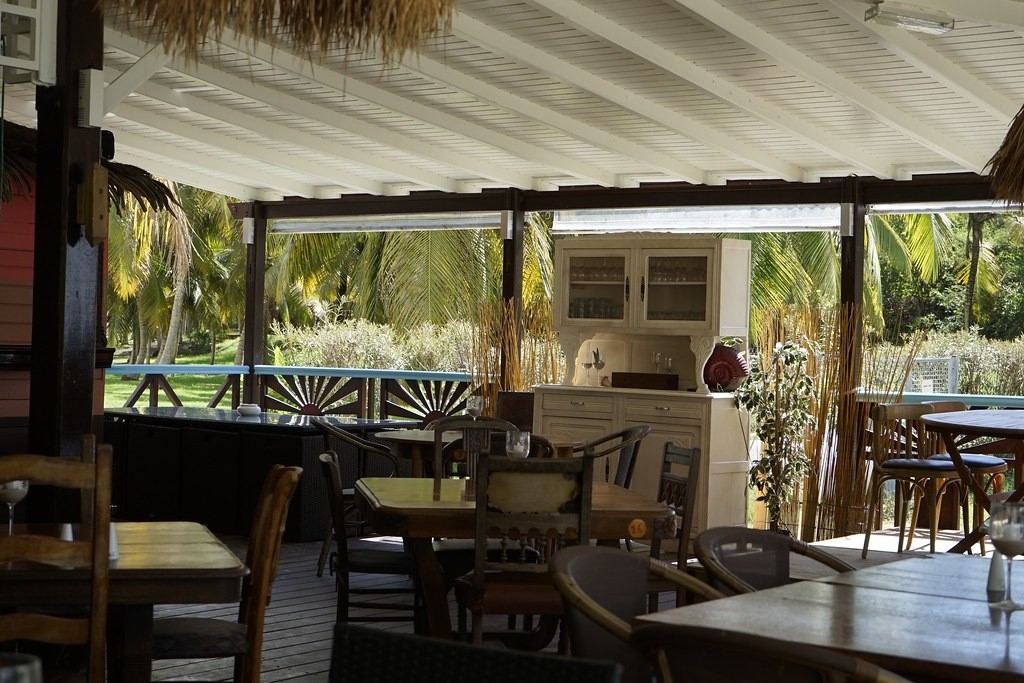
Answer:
[530,235,752,556]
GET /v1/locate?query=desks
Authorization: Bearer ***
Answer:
[919,409,1024,554]
[104,406,423,429]
[634,552,1024,683]
[0,522,250,683]
[354,478,676,641]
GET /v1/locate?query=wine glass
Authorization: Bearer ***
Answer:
[0,479,30,536]
[988,502,1024,610]
[594,352,606,387]
[581,352,592,386]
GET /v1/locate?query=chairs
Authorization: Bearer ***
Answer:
[573,425,651,488]
[861,401,1007,559]
[309,417,400,578]
[549,545,729,683]
[152,465,303,683]
[0,434,113,683]
[456,446,594,653]
[648,442,701,613]
[435,415,555,477]
[319,451,421,635]
[693,527,856,594]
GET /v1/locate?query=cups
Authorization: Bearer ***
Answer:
[648,311,704,321]
[466,397,484,422]
[506,431,530,458]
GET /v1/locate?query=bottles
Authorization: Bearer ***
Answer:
[649,268,706,281]
[108,522,120,560]
[987,550,1006,592]
[236,402,261,417]
[569,297,622,318]
[571,266,623,280]
[650,352,680,374]
[60,523,73,542]
